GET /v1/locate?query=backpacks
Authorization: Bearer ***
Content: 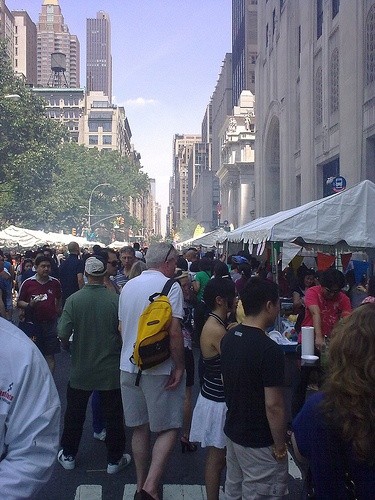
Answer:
[130,278,181,371]
[175,270,194,301]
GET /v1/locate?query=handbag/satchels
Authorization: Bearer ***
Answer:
[19,305,44,354]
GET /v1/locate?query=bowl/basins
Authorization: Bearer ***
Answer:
[278,342,298,352]
[301,355,319,363]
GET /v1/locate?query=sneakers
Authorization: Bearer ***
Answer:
[106,453,131,474]
[58,449,76,470]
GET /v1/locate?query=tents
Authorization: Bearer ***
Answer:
[219,179,375,295]
[49,232,105,247]
[108,241,129,251]
[0,225,61,250]
[175,227,229,252]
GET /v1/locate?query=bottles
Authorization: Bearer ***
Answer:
[320,335,329,366]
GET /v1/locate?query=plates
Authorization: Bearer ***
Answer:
[269,331,282,342]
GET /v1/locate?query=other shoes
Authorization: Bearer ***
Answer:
[134,489,155,500]
[94,429,106,441]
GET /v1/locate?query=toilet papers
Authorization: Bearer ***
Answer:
[301,326,315,356]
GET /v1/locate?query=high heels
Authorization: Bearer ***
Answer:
[180,436,197,454]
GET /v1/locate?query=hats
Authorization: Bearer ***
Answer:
[85,255,108,277]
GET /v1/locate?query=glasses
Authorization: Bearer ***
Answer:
[164,244,175,265]
[107,260,121,268]
[324,287,340,295]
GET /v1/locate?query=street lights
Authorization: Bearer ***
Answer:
[79,184,123,238]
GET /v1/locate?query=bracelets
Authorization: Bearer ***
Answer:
[274,442,287,458]
[28,303,30,308]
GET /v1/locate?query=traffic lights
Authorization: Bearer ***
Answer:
[121,217,125,225]
[118,218,121,225]
[71,228,77,236]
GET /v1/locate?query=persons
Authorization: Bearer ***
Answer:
[189,278,239,500]
[80,242,375,349]
[117,242,187,500]
[92,248,121,442]
[298,268,351,353]
[219,277,288,500]
[0,315,62,500]
[60,241,86,352]
[172,269,200,453]
[19,256,65,379]
[290,302,375,500]
[57,254,134,476]
[0,246,65,323]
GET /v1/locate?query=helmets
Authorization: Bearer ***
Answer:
[232,255,248,264]
